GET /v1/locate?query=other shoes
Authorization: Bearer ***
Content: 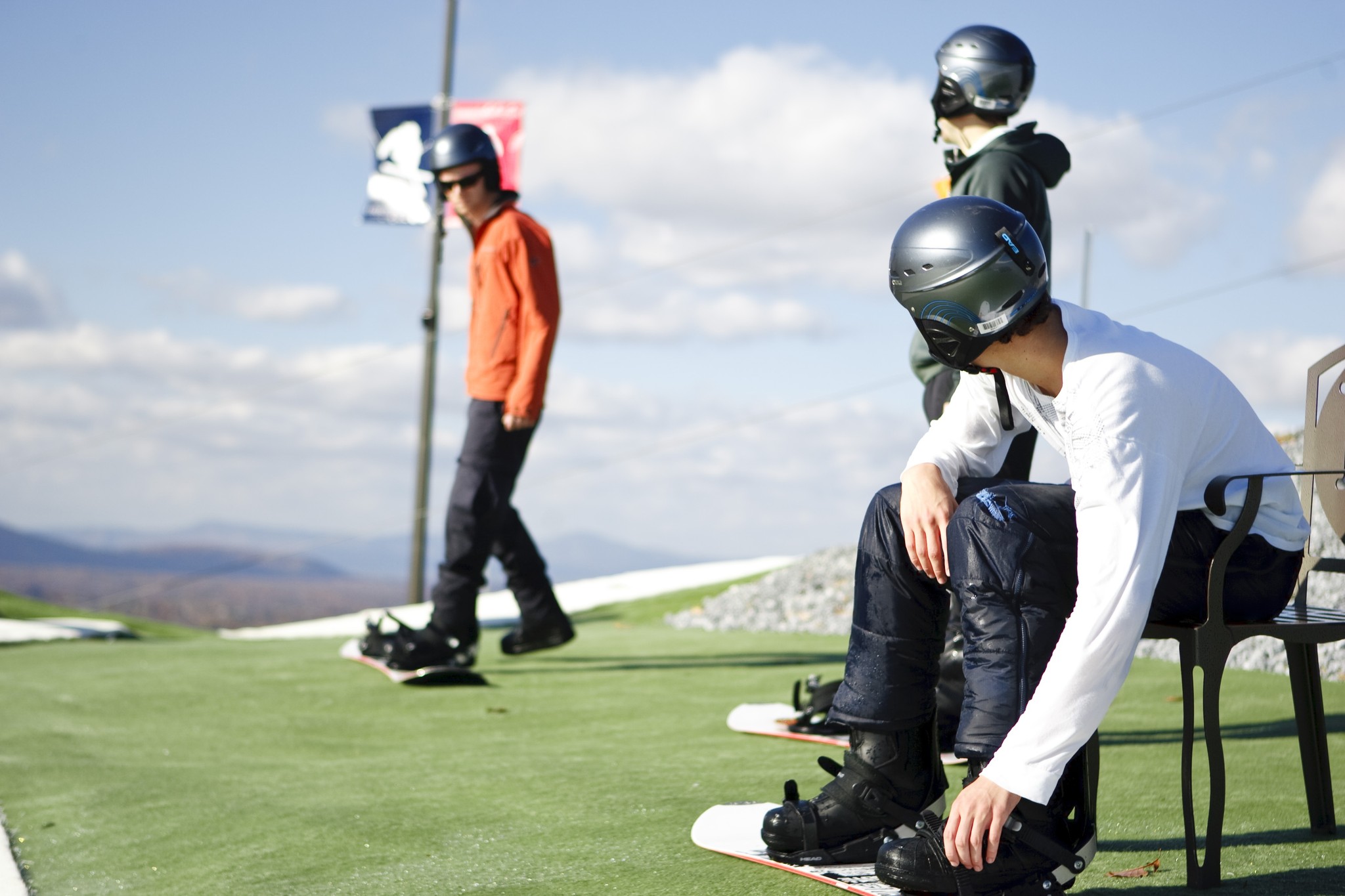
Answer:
[500,613,575,654]
[875,753,1087,896]
[387,624,479,671]
[760,700,938,851]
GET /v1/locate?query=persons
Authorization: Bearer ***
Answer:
[359,125,575,669]
[800,24,1070,737]
[759,197,1309,895]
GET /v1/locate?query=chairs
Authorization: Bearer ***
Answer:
[1087,346,1345,896]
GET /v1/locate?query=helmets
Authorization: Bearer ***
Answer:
[889,195,1049,368]
[431,123,498,172]
[935,25,1035,116]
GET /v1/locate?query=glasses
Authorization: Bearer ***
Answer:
[437,166,491,192]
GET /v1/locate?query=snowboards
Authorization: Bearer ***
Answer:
[340,637,492,687]
[724,703,969,765]
[690,802,900,896]
